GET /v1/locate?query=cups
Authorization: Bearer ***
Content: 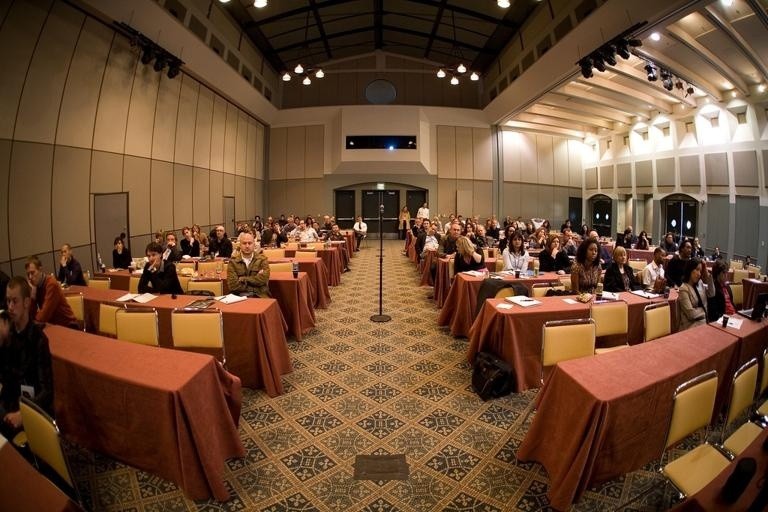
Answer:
[293,271,299,279]
[515,271,520,279]
[596,283,605,299]
[101,264,106,273]
[722,315,729,327]
[215,263,224,274]
[130,262,135,271]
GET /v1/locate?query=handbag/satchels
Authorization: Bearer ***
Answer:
[655,276,668,294]
[472,352,514,401]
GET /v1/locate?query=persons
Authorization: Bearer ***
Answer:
[499,216,570,272]
[157,223,233,262]
[227,234,271,298]
[417,203,429,218]
[25,256,80,331]
[1,271,53,441]
[139,242,184,295]
[353,216,367,251]
[637,231,737,332]
[235,214,344,248]
[57,244,87,286]
[561,219,637,295]
[412,214,500,274]
[744,256,753,270]
[399,206,410,240]
[113,238,132,269]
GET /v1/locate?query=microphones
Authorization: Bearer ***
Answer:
[380,204,384,216]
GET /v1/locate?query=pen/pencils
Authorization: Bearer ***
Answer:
[520,299,533,302]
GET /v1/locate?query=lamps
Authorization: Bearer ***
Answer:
[282,0,325,85]
[577,21,650,79]
[635,53,710,98]
[438,2,479,85]
[114,21,186,78]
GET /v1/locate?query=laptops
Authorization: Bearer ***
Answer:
[737,294,768,319]
